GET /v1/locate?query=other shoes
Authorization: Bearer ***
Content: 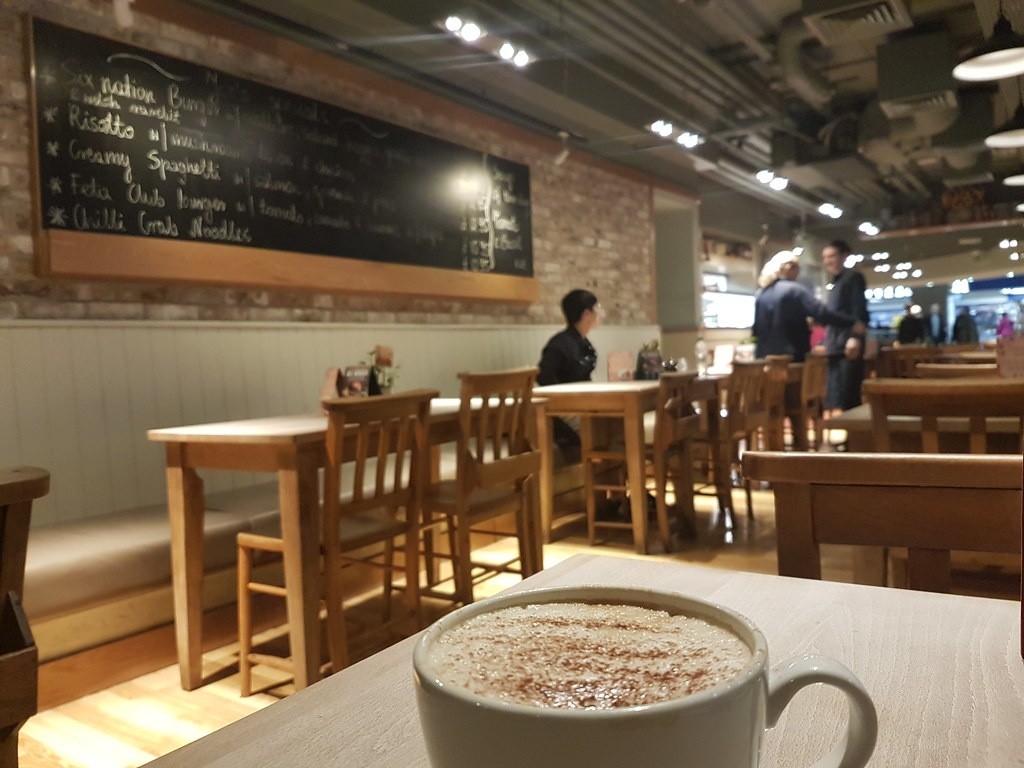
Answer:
[608,491,660,514]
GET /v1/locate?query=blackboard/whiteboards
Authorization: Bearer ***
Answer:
[25,13,539,303]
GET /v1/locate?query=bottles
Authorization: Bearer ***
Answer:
[694,336,708,379]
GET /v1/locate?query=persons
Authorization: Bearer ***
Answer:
[995,312,1014,337]
[893,303,946,348]
[820,240,869,414]
[950,306,978,343]
[538,288,670,514]
[754,250,868,447]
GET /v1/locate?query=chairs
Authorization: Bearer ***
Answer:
[605,351,828,554]
[232,365,541,698]
[742,343,1024,580]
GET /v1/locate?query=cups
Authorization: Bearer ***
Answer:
[412,583,878,768]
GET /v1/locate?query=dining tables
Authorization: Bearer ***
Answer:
[142,550,1024,768]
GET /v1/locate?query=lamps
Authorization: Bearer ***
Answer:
[953,6,1024,220]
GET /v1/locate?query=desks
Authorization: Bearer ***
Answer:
[145,398,555,691]
[530,379,675,555]
[824,395,1019,459]
